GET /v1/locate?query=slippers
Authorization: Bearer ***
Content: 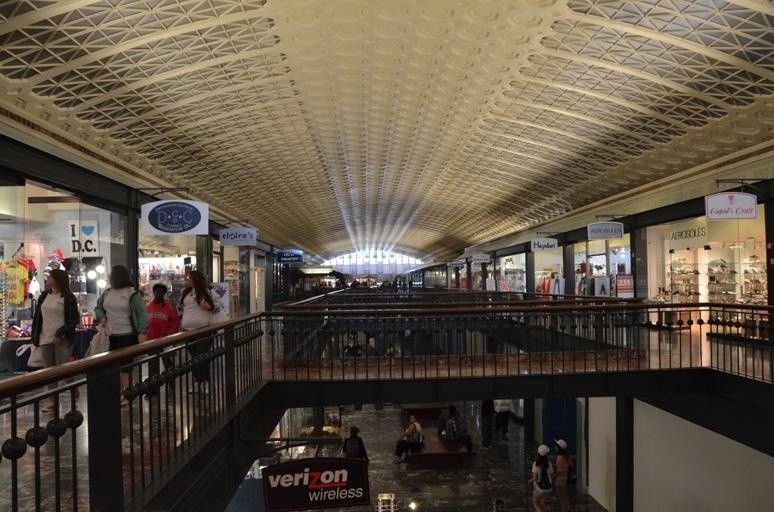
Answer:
[537,463,553,490]
[564,459,578,485]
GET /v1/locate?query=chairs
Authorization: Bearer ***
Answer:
[42,402,60,416]
[189,380,210,394]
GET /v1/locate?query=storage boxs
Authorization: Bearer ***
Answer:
[400,402,468,468]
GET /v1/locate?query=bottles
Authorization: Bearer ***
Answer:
[553,438,568,448]
[538,444,550,455]
[42,260,60,277]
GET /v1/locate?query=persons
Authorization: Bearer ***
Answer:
[392,415,423,464]
[30,269,80,413]
[145,283,181,400]
[528,439,577,512]
[177,271,224,395]
[437,405,476,456]
[496,410,511,441]
[343,426,368,461]
[93,265,148,407]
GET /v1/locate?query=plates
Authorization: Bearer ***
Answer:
[666,259,766,298]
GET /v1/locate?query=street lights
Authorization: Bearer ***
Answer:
[209,289,228,325]
[16,343,49,367]
